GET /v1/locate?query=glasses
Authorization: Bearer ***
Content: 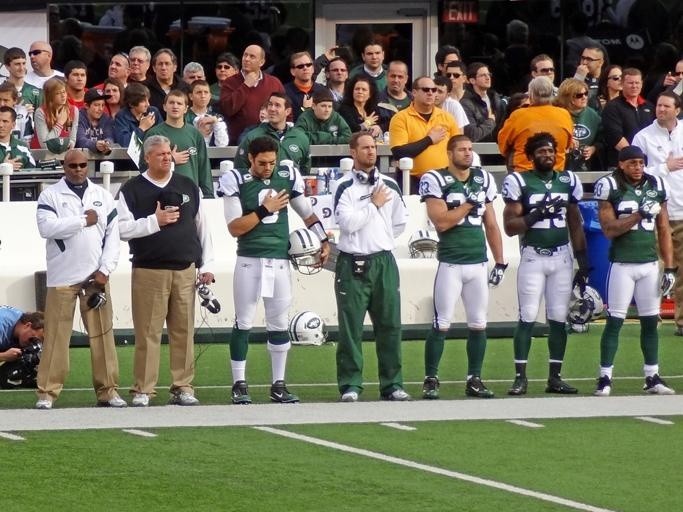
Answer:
[540,67,554,73]
[580,56,601,61]
[446,72,460,78]
[29,49,49,56]
[69,163,87,169]
[217,65,230,69]
[295,63,312,69]
[608,75,619,81]
[575,91,588,98]
[415,88,437,92]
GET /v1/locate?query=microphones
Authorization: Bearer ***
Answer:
[202,278,215,287]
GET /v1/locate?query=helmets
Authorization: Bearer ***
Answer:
[567,286,603,324]
[409,229,438,258]
[289,311,329,346]
[287,229,323,275]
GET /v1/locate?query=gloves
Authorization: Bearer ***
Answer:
[660,266,679,296]
[467,191,486,204]
[572,266,594,297]
[532,192,564,221]
[639,196,656,218]
[489,262,509,288]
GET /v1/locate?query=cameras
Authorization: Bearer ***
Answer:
[573,145,584,161]
[336,48,351,55]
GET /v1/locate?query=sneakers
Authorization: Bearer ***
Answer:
[132,393,149,406]
[98,395,127,407]
[271,379,299,403]
[593,375,613,396]
[545,377,578,393]
[342,392,358,402]
[172,393,199,405]
[35,400,52,409]
[230,381,252,404]
[464,374,493,398]
[643,373,675,394]
[508,377,527,395]
[382,389,410,401]
[423,377,440,399]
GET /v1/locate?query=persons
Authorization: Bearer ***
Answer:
[0,305,44,364]
[114,135,215,406]
[631,87,683,336]
[219,136,330,404]
[1,40,683,199]
[332,130,412,401]
[501,132,589,395]
[418,135,508,399]
[34,149,128,409]
[593,146,679,397]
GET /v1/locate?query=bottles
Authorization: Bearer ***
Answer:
[316,169,325,195]
[327,169,337,196]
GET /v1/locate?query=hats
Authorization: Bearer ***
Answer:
[84,88,112,101]
[154,191,183,225]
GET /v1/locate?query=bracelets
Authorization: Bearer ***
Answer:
[308,220,328,243]
[254,205,269,221]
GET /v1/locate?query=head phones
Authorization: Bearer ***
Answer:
[198,286,221,313]
[79,279,107,311]
